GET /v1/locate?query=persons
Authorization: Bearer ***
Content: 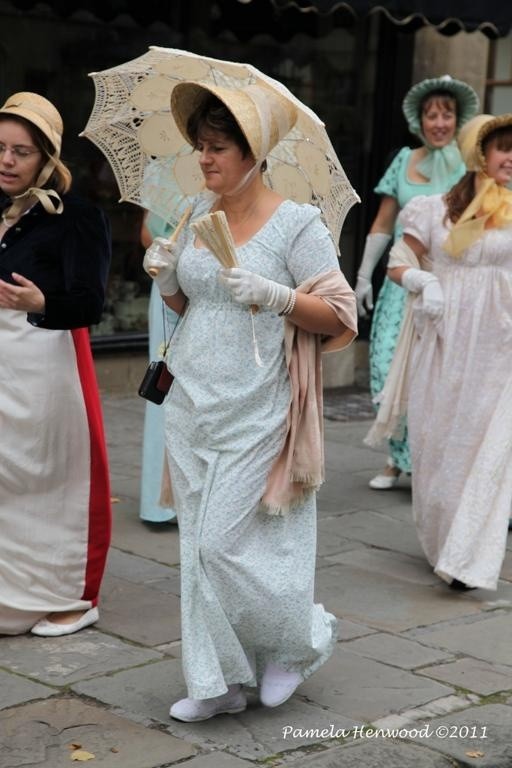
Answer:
[355,74,482,492]
[140,165,194,525]
[387,112,512,591]
[0,83,112,641]
[141,73,359,725]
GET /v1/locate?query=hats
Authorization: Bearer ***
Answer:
[0,91,63,157]
[402,75,480,136]
[458,113,512,172]
[170,80,298,163]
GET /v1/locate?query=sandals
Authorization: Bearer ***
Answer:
[369,456,401,489]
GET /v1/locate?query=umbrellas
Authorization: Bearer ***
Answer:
[80,44,365,281]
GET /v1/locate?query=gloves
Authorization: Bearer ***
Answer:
[220,268,290,314]
[355,233,392,320]
[401,268,445,326]
[143,236,179,296]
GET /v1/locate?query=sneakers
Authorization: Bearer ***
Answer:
[31,606,99,636]
[259,656,304,708]
[169,684,247,723]
